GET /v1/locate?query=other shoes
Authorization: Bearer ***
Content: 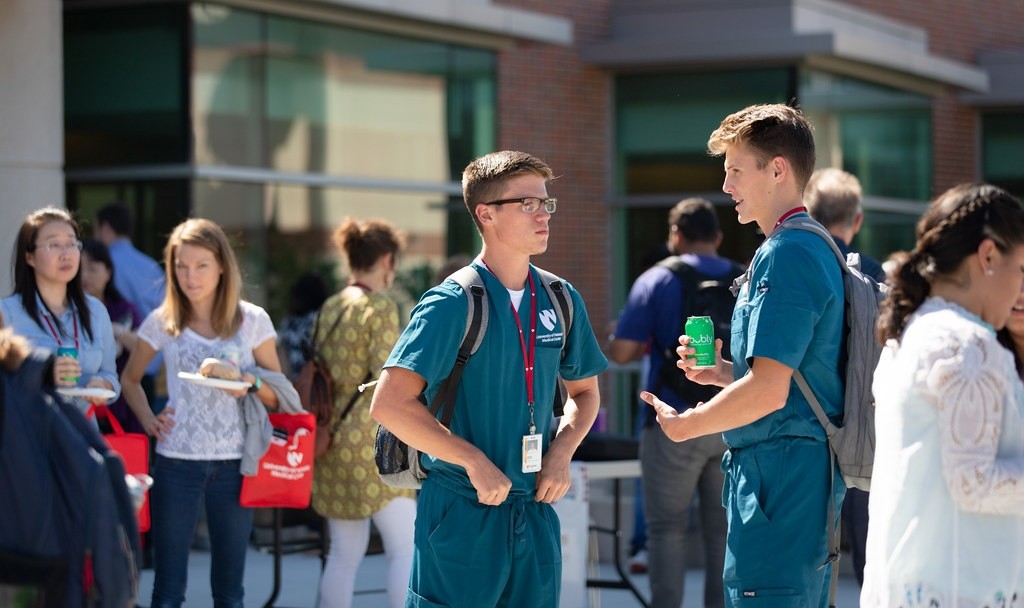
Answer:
[628,548,648,575]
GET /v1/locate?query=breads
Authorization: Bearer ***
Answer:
[199,357,241,380]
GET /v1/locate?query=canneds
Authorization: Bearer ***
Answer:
[685,315,717,370]
[56,344,78,383]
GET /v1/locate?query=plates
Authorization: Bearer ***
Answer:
[57,387,115,398]
[178,372,252,390]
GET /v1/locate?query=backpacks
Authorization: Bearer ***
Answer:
[374,263,573,489]
[641,255,747,414]
[0,348,143,608]
[292,291,374,461]
[729,218,892,608]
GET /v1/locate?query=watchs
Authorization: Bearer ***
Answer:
[247,375,262,395]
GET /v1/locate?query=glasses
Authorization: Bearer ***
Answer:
[36,239,83,253]
[485,197,557,213]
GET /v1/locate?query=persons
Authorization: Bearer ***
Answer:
[640,105,847,608]
[606,197,749,608]
[527,441,537,454]
[802,169,1024,608]
[369,151,609,608]
[0,201,475,608]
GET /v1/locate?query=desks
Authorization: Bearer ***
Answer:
[573,457,647,608]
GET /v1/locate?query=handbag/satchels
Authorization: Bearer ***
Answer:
[86,401,151,533]
[240,411,316,508]
